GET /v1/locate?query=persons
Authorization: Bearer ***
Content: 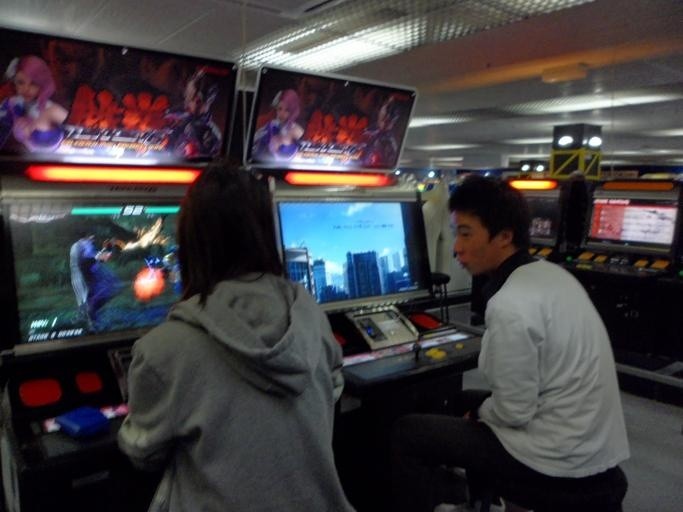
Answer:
[251,90,310,163]
[155,70,223,159]
[70,227,125,335]
[357,176,631,512]
[116,165,355,511]
[4,56,68,155]
[344,93,412,167]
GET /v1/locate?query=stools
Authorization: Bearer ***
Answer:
[522,466,628,511]
[430,272,451,324]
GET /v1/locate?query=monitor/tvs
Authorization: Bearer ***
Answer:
[528,202,558,239]
[587,198,679,253]
[1,195,186,343]
[278,201,424,304]
